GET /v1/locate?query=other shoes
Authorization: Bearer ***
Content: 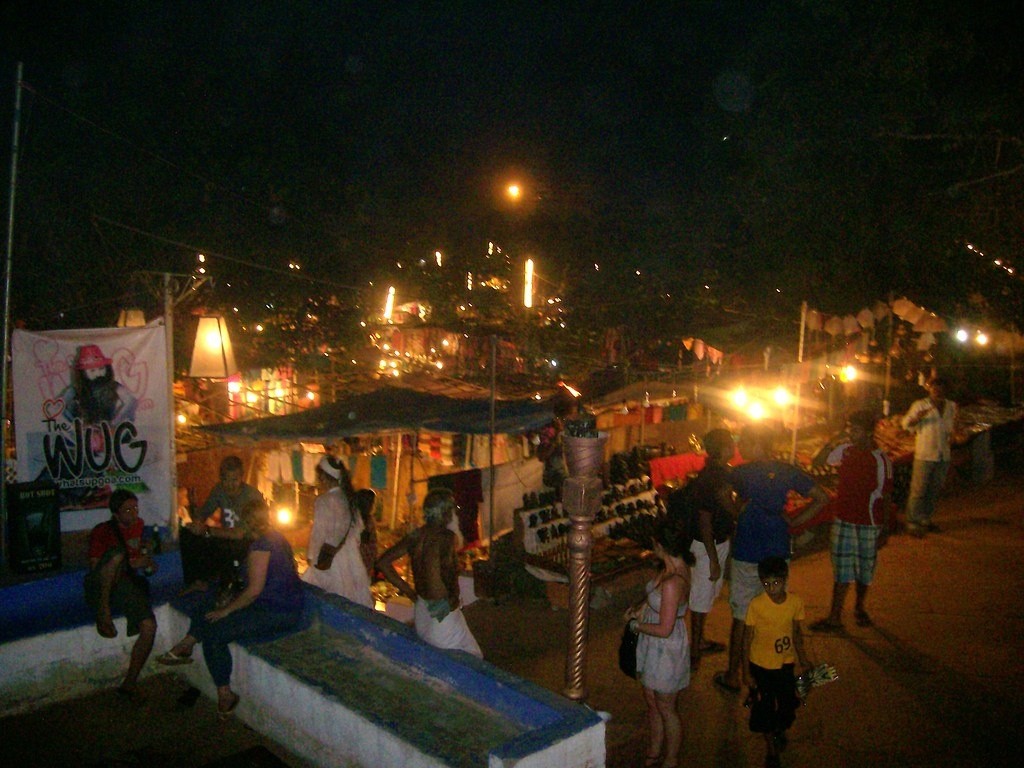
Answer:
[774,729,787,752]
[700,640,727,653]
[908,522,923,538]
[689,652,702,666]
[919,518,937,532]
[124,681,147,703]
[765,750,781,768]
[96,610,118,637]
[714,673,741,692]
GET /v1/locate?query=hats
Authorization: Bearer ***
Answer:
[925,367,944,387]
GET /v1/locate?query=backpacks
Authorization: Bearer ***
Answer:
[667,476,699,551]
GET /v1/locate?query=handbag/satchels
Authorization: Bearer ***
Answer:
[314,543,337,571]
[618,618,640,680]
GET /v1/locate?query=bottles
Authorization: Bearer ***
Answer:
[795,676,807,707]
[231,556,245,590]
[152,524,161,552]
[139,546,156,577]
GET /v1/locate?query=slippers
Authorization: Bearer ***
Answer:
[156,650,194,665]
[218,694,241,723]
[808,616,844,632]
[855,610,870,627]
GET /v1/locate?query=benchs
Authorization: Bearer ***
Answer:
[0,558,607,768]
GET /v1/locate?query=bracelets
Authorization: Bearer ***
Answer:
[629,620,638,631]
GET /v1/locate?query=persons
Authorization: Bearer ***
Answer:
[622,518,696,768]
[378,488,484,661]
[901,376,968,537]
[714,423,830,694]
[50,345,137,427]
[178,456,269,594]
[155,499,304,725]
[84,489,157,700]
[351,489,376,578]
[686,429,732,671]
[301,455,373,612]
[742,556,813,768]
[809,409,893,630]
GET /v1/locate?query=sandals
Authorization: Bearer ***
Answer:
[641,753,663,768]
